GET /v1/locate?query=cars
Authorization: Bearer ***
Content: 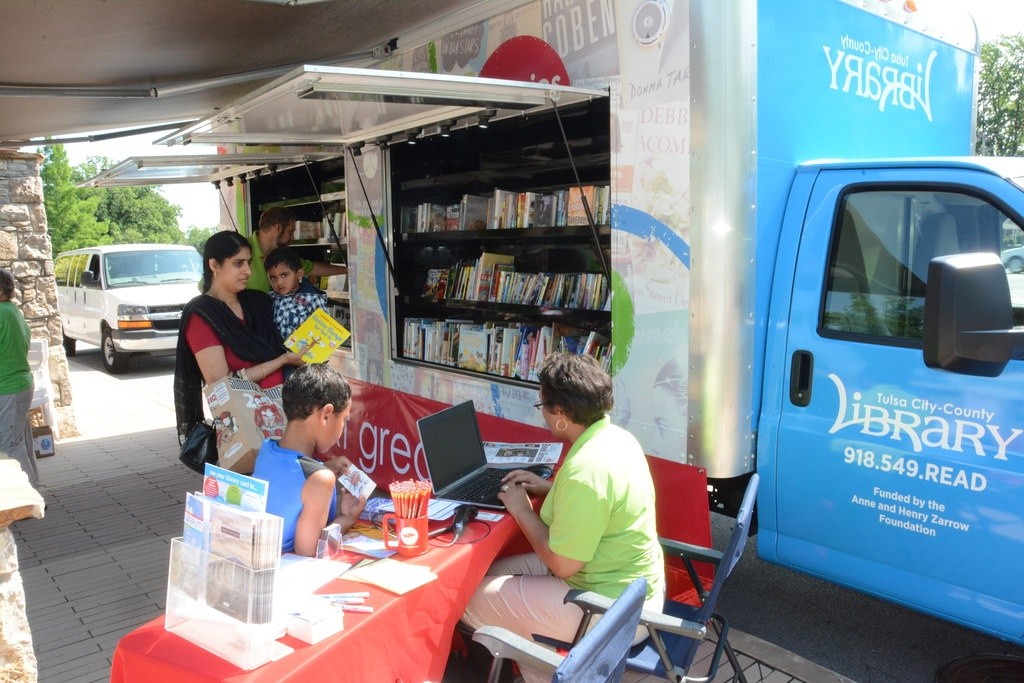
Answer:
[1001,246,1024,274]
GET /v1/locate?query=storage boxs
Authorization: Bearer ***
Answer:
[31,426,55,458]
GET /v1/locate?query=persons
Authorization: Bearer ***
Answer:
[252,362,367,557]
[198,207,348,294]
[0,268,39,488]
[460,352,666,683]
[263,246,328,381]
[174,230,306,475]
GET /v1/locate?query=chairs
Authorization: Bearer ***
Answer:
[825,210,892,337]
[532,472,760,683]
[472,575,647,683]
[27,338,60,441]
[912,212,961,336]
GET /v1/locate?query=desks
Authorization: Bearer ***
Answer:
[116,492,544,683]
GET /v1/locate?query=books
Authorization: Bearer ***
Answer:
[403,315,612,384]
[422,252,607,310]
[416,186,611,233]
[282,308,351,364]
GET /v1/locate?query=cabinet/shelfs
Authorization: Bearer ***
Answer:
[255,189,352,349]
[394,150,613,388]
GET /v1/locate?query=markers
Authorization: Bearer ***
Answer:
[320,592,369,598]
[333,603,373,613]
[328,598,365,604]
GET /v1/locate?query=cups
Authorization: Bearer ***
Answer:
[382,513,429,557]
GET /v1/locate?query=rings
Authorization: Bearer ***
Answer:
[503,485,508,491]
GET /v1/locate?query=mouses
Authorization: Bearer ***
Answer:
[526,464,553,478]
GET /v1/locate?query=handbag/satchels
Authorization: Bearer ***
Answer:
[204,370,288,475]
[180,419,219,475]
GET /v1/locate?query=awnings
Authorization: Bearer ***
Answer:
[71,152,348,266]
[152,64,609,292]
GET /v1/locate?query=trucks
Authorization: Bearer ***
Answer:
[0,1,1024,648]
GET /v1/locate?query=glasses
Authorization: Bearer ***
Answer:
[533,399,550,411]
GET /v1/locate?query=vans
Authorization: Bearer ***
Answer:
[53,243,204,374]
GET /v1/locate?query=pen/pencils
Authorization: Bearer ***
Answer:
[389,478,431,518]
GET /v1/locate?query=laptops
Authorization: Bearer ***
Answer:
[417,400,552,509]
